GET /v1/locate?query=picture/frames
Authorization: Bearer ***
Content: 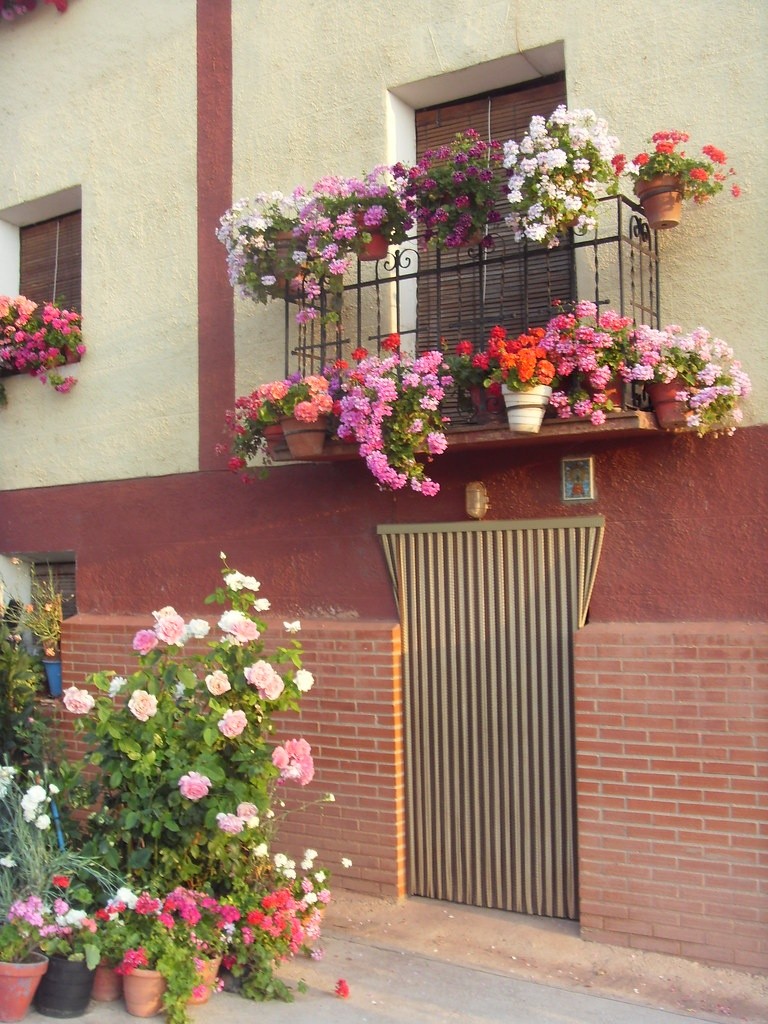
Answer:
[562,457,594,500]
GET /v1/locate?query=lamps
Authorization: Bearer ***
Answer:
[465,481,493,520]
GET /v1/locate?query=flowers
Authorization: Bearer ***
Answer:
[0,558,353,1024]
[213,104,751,497]
[0,296,85,409]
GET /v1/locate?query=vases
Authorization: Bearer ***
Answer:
[633,170,683,228]
[501,383,553,432]
[280,414,328,458]
[188,956,223,1005]
[91,956,123,1001]
[272,232,303,277]
[0,951,49,1022]
[35,948,95,1019]
[124,968,167,1018]
[357,212,388,261]
[42,660,62,697]
[644,378,696,429]
